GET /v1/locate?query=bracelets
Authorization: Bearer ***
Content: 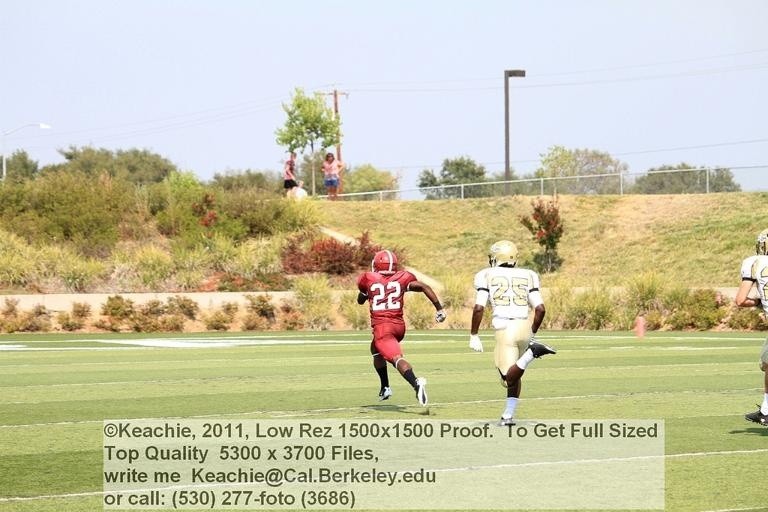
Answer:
[434,301,442,310]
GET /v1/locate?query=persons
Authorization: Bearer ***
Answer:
[469,240,556,427]
[357,250,446,407]
[735,228,768,427]
[320,153,344,202]
[295,180,307,200]
[283,152,298,202]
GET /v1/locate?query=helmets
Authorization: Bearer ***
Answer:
[371,250,397,275]
[756,228,768,255]
[488,240,518,267]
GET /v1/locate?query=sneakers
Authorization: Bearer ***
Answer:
[499,416,513,426]
[379,386,391,402]
[744,410,768,426]
[525,339,556,359]
[413,377,428,407]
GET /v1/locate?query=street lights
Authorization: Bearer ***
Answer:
[0,121,53,178]
[502,67,526,194]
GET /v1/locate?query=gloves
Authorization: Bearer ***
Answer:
[435,310,446,323]
[469,333,484,353]
